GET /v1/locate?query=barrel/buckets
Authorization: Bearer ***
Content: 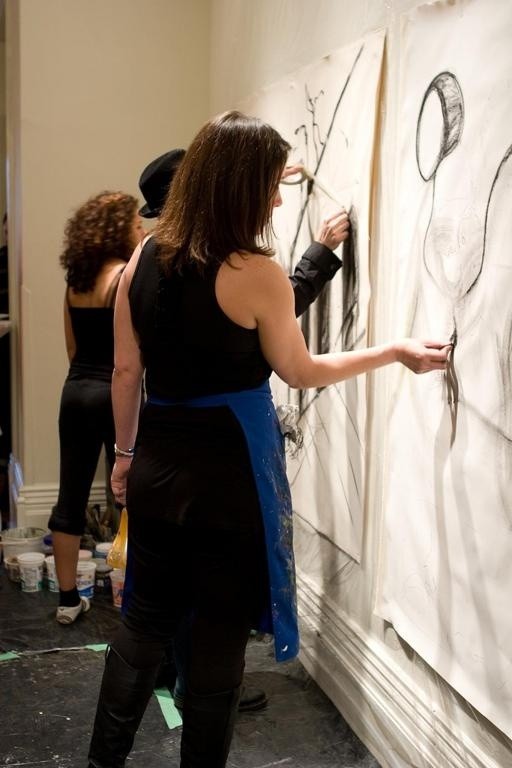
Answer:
[78,550,92,559]
[93,540,115,557]
[75,561,97,599]
[44,555,61,594]
[0,526,52,569]
[15,553,47,593]
[109,570,128,608]
[89,557,114,593]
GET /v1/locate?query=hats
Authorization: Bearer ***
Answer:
[135,148,189,218]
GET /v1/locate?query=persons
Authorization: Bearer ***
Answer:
[134,145,355,716]
[45,188,151,627]
[80,108,455,768]
[0,211,14,514]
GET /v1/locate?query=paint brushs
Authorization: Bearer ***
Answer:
[85,502,112,542]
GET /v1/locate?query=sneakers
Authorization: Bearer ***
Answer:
[54,594,92,626]
[169,677,270,714]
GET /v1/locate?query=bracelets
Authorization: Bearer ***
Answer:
[113,441,134,457]
